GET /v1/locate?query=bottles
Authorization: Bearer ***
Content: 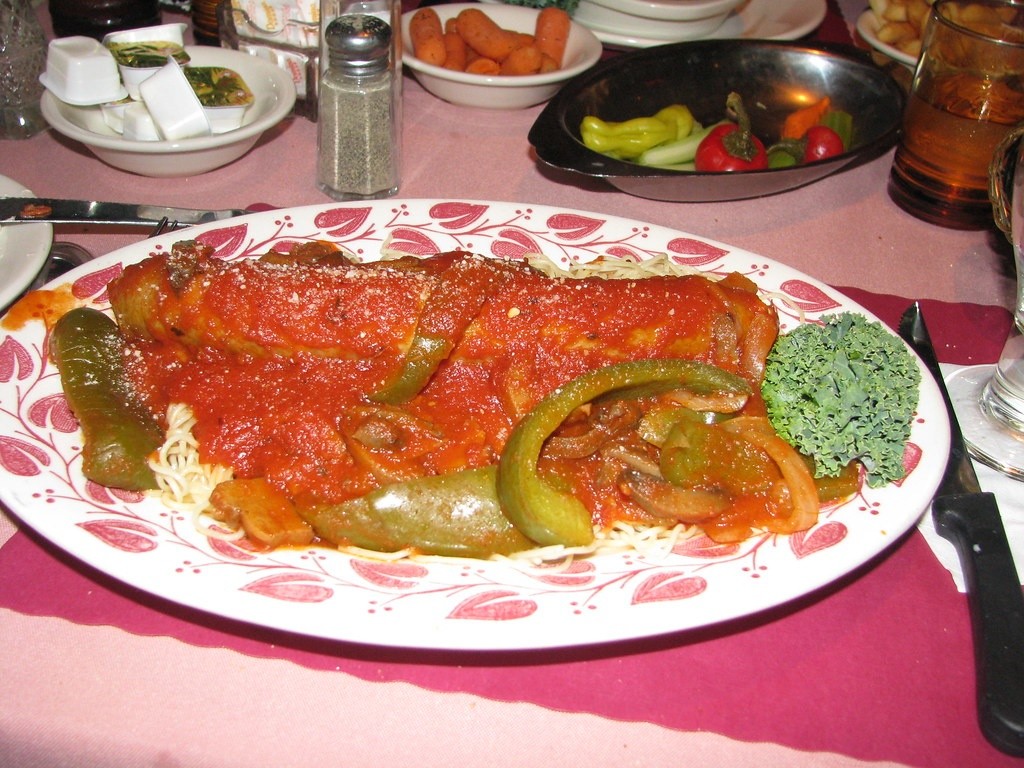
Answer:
[319,15,400,200]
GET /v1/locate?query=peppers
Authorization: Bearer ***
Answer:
[42,251,860,562]
[579,91,844,175]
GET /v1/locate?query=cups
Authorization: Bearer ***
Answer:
[888,0,1024,230]
[0,0,51,140]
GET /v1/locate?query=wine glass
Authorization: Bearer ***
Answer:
[944,138,1024,482]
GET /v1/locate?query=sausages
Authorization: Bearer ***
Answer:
[409,6,570,75]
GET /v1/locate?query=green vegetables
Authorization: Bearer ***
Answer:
[760,310,924,489]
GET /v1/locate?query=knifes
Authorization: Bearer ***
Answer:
[0,197,257,226]
[0,197,951,650]
[897,300,1024,756]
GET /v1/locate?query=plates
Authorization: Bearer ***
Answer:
[0,174,53,310]
[481,0,827,54]
[854,7,918,76]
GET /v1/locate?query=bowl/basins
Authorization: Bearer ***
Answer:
[528,38,905,201]
[573,0,745,39]
[39,45,298,179]
[401,3,603,109]
